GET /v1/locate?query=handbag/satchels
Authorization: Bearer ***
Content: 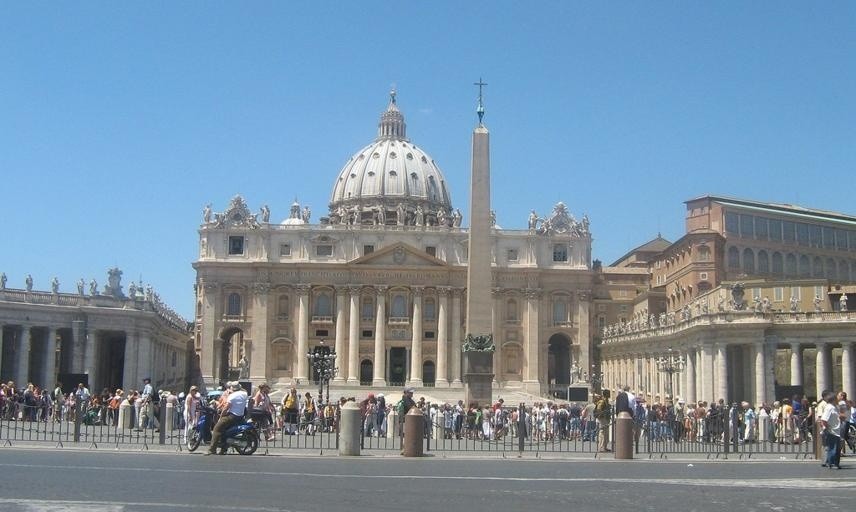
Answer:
[307,397,316,414]
[777,412,784,425]
[820,429,834,451]
[593,398,610,418]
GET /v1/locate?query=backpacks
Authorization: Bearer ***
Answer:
[49,390,57,402]
[152,386,160,406]
[397,398,407,413]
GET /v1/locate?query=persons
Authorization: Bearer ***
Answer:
[813,294,825,311]
[238,355,250,378]
[754,296,763,312]
[581,213,591,233]
[52,276,62,293]
[78,278,87,294]
[338,203,348,226]
[414,206,425,226]
[603,296,708,340]
[790,294,799,312]
[396,202,407,227]
[0,272,7,290]
[452,206,463,227]
[259,202,270,222]
[491,209,496,229]
[128,281,137,300]
[26,274,34,291]
[839,291,850,312]
[570,360,583,382]
[764,294,772,311]
[353,204,363,224]
[437,206,447,226]
[145,284,188,336]
[90,278,98,295]
[302,205,312,224]
[527,208,539,235]
[201,204,211,223]
[376,204,385,225]
[718,294,726,312]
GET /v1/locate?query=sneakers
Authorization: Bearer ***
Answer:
[203,447,227,456]
[132,427,160,432]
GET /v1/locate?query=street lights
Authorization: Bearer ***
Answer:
[655,346,685,407]
[306,338,339,411]
[583,363,604,403]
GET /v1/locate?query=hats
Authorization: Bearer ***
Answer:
[230,380,243,392]
[115,388,124,396]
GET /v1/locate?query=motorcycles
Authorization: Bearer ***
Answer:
[186,402,261,456]
